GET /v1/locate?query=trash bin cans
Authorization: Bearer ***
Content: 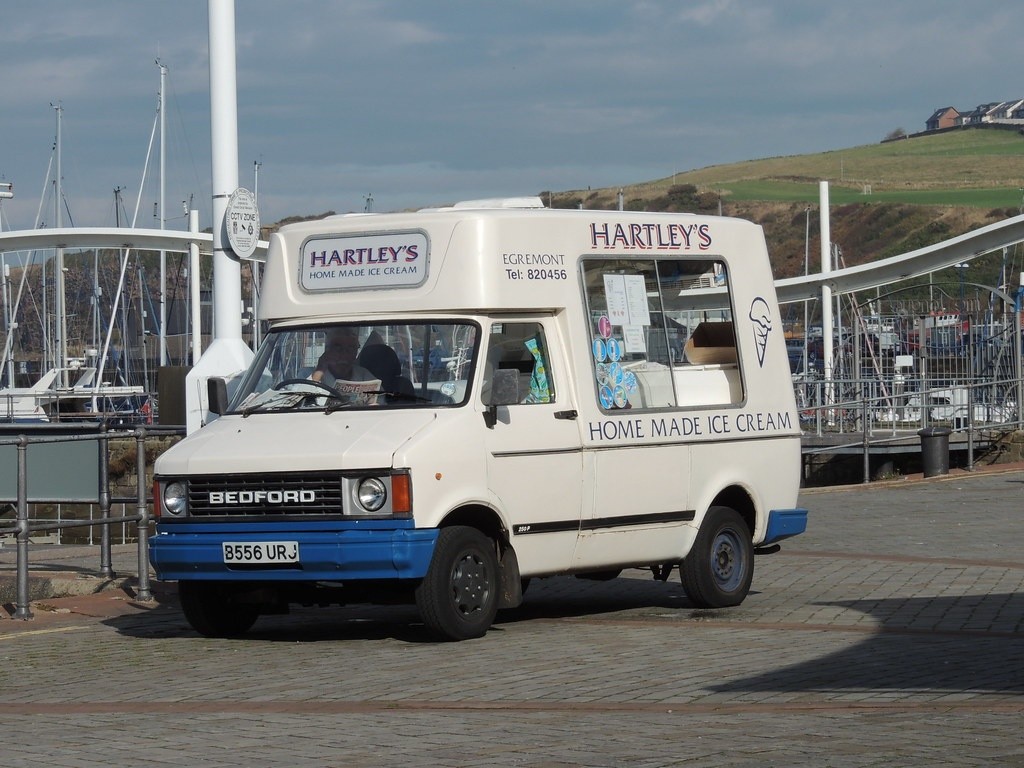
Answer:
[917,426,953,478]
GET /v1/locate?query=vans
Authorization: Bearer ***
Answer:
[149,197,814,638]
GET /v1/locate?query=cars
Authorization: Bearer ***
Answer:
[785,336,805,358]
[807,337,862,360]
[843,331,1008,358]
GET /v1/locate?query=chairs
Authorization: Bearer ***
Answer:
[357,344,418,404]
[481,354,520,406]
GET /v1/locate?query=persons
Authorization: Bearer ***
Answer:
[310,328,401,384]
